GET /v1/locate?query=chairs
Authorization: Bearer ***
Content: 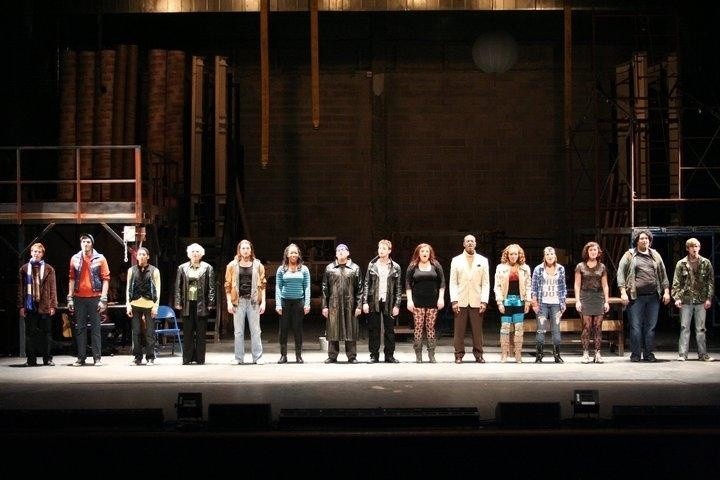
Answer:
[154,305,184,358]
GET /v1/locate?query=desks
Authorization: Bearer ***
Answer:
[47,305,140,358]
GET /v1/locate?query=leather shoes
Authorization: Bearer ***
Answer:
[324,358,337,364]
[477,355,486,363]
[367,355,379,364]
[455,356,463,365]
[384,356,399,364]
[348,358,358,364]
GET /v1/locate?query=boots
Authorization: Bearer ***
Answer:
[594,349,605,364]
[426,339,437,364]
[581,349,591,365]
[553,344,565,364]
[534,342,544,365]
[412,341,423,363]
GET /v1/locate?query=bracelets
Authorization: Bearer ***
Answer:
[604,301,609,303]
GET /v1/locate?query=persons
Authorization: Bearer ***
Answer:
[225,240,267,366]
[363,240,403,364]
[17,233,161,366]
[174,243,217,365]
[531,246,567,364]
[449,234,490,363]
[671,238,715,361]
[275,243,312,364]
[574,242,611,363]
[494,243,531,363]
[617,229,671,362]
[405,243,446,363]
[321,244,364,364]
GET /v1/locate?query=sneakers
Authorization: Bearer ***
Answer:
[44,359,55,367]
[183,359,193,365]
[297,356,303,364]
[499,355,509,363]
[676,353,687,362]
[197,358,204,365]
[698,352,715,362]
[130,358,142,367]
[630,353,642,363]
[255,356,264,366]
[93,358,102,367]
[24,361,36,367]
[515,354,522,364]
[145,358,155,366]
[278,355,288,365]
[233,359,244,365]
[645,352,657,363]
[72,359,86,366]
